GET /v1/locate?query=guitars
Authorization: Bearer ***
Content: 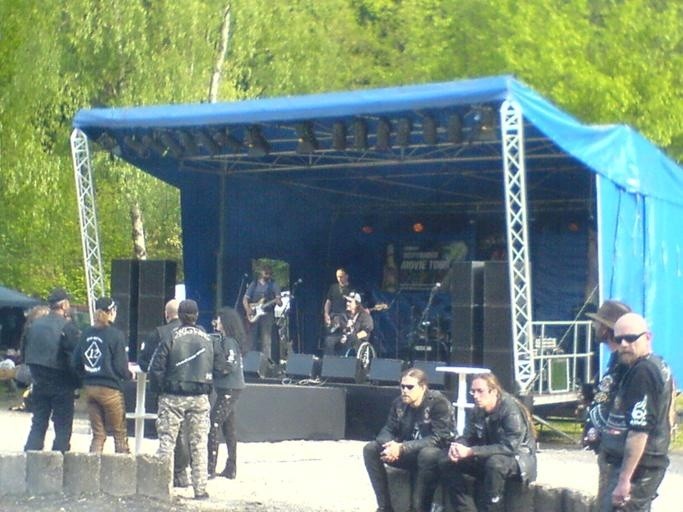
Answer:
[325,302,389,334]
[247,294,295,324]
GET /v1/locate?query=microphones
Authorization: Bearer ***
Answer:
[293,278,303,285]
[239,273,249,279]
[433,282,441,291]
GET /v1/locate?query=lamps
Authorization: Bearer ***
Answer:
[95,100,497,159]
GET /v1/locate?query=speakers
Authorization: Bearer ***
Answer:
[109,258,137,360]
[320,354,363,383]
[450,260,484,367]
[368,356,410,384]
[411,360,447,388]
[286,353,321,378]
[242,350,275,378]
[481,261,532,394]
[136,259,177,371]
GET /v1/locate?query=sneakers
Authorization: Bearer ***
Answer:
[195,489,209,500]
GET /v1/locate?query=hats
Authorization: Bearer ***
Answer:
[343,291,361,302]
[48,288,70,301]
[261,265,274,274]
[95,296,115,312]
[179,299,198,313]
[585,300,631,330]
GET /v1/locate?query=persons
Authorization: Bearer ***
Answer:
[437,370,538,512]
[147,298,226,501]
[593,310,675,512]
[361,366,459,512]
[323,265,372,328]
[243,262,283,358]
[582,299,633,455]
[19,288,80,453]
[321,290,374,357]
[67,296,138,454]
[135,297,190,489]
[207,305,246,481]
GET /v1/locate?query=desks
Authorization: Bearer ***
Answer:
[125,364,158,455]
[434,366,491,438]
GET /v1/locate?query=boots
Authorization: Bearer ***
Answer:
[208,459,237,481]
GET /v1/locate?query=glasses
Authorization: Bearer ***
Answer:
[468,389,491,396]
[400,384,415,389]
[614,332,644,344]
